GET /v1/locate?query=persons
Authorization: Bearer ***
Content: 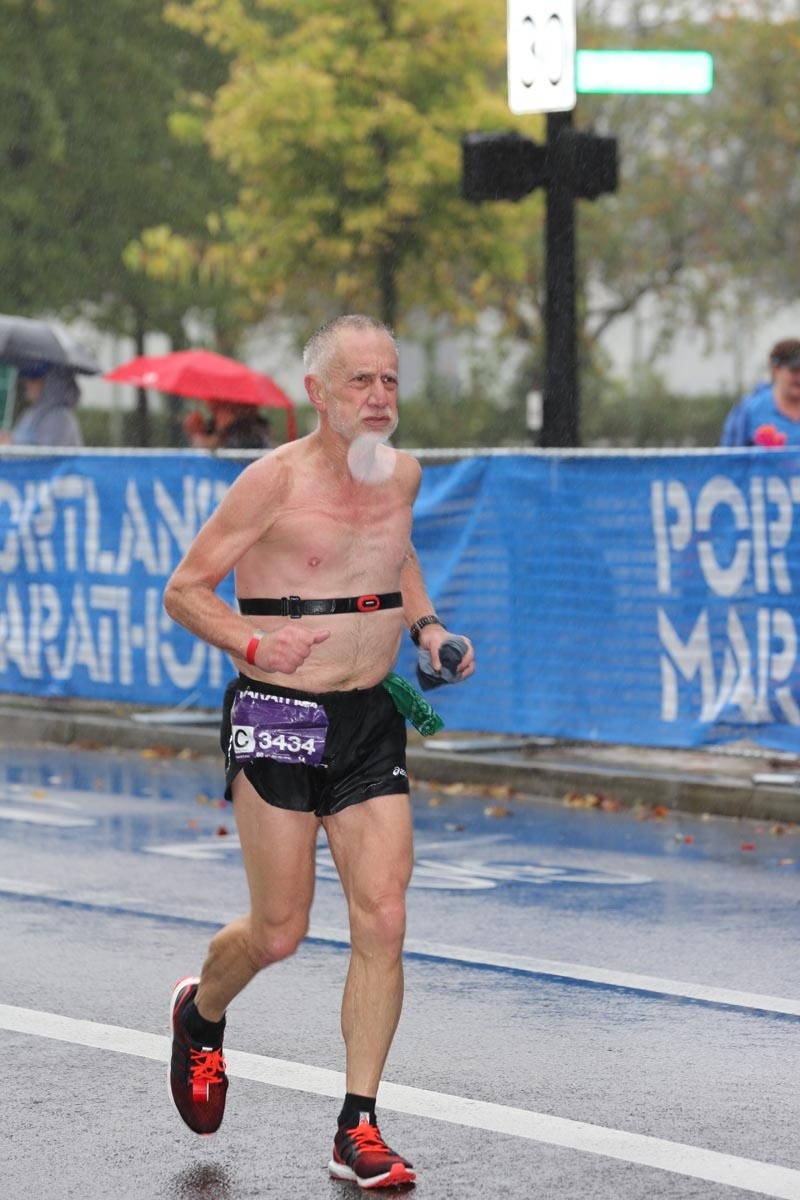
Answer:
[721,340,800,448]
[0,362,272,448]
[165,316,475,1187]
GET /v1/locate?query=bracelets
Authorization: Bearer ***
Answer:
[408,616,447,648]
[245,629,267,664]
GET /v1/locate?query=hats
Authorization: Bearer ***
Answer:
[770,339,800,370]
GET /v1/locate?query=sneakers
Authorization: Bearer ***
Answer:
[326,1108,416,1188]
[166,974,229,1138]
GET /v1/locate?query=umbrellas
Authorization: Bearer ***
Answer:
[1,312,299,443]
